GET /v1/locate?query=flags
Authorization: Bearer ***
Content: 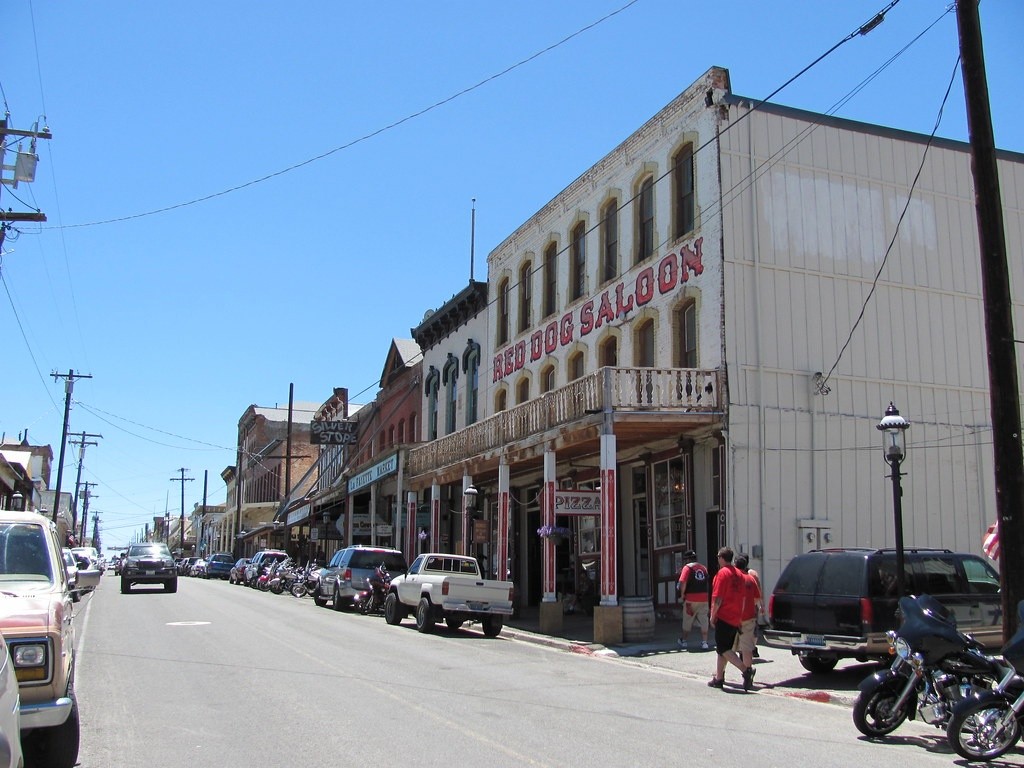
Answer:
[983,517,1000,562]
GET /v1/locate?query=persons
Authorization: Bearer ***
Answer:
[677,550,710,649]
[733,556,761,668]
[737,553,764,657]
[708,547,757,692]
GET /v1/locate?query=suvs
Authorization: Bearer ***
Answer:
[314,545,408,611]
[244,551,289,587]
[121,543,178,593]
[203,553,235,580]
[761,545,1006,671]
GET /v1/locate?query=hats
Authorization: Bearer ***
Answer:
[683,550,697,559]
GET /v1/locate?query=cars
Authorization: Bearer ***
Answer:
[0,632,25,768]
[0,510,101,730]
[61,546,106,602]
[229,558,254,586]
[108,564,116,570]
[174,557,205,578]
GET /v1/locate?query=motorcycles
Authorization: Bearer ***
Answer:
[853,594,1024,762]
[250,557,317,598]
[355,561,393,615]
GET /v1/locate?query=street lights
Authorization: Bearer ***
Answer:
[874,400,911,597]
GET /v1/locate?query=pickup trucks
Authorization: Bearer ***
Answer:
[384,553,514,637]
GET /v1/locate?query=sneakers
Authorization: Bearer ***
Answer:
[677,638,687,647]
[741,667,756,692]
[700,641,708,649]
[708,677,724,688]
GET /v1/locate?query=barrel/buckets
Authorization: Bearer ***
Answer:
[618,597,655,642]
